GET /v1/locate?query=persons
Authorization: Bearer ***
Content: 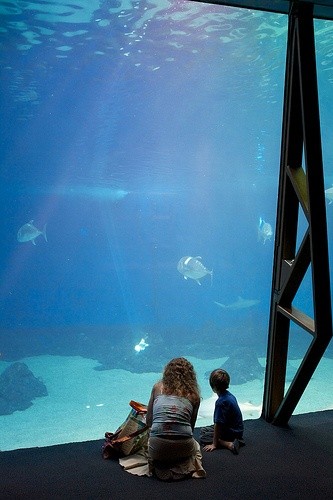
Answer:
[200,369,245,455]
[145,357,206,482]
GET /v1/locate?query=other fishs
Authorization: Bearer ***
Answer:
[16,219,48,246]
[178,255,212,285]
[258,217,273,245]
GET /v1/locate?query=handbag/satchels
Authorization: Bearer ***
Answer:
[101,432,122,460]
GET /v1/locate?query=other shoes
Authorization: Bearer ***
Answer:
[231,439,240,455]
